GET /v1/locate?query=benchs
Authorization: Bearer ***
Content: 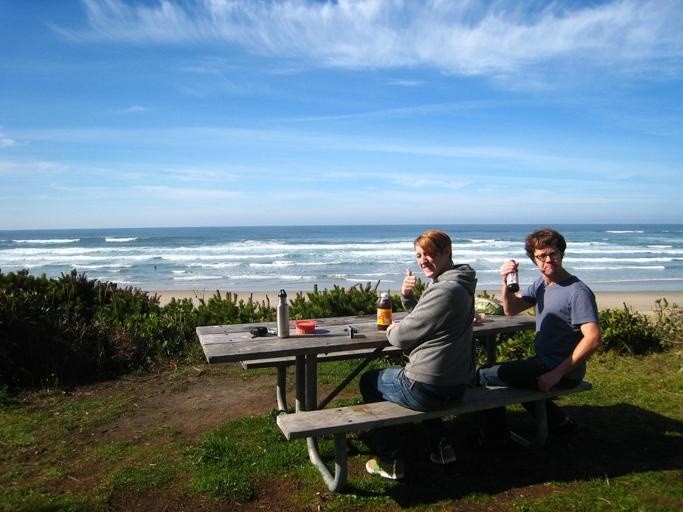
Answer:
[276,380,592,492]
[241,346,414,415]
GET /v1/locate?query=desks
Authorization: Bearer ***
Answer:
[195,312,536,494]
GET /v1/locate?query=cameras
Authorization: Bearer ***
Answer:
[250,327,268,337]
[344,324,360,339]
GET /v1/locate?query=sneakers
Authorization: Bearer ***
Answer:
[365,451,410,480]
[432,440,457,464]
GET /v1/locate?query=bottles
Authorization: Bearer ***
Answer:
[507,260,519,293]
[376,293,392,331]
[276,289,289,338]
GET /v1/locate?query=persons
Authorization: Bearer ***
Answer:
[475,225,604,436]
[359,228,479,481]
